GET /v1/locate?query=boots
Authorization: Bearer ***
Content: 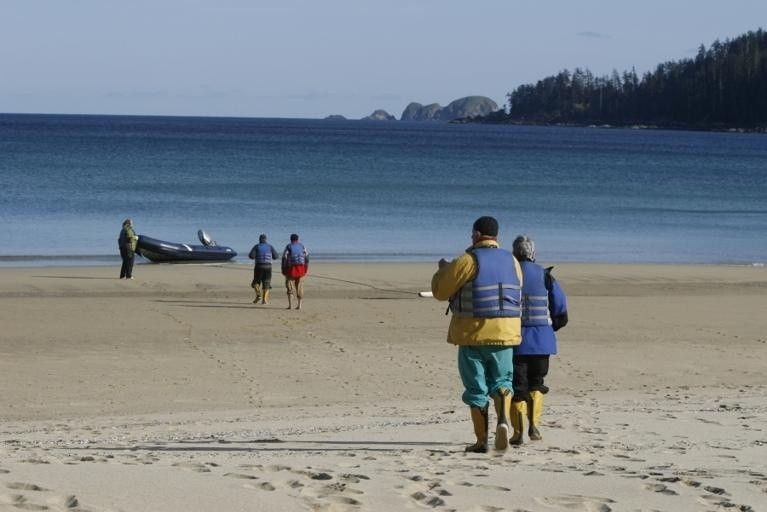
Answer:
[494,386,512,451]
[509,400,528,445]
[252,283,262,304]
[262,288,269,304]
[526,390,544,441]
[465,401,490,452]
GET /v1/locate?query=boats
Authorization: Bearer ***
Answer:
[135,229,238,262]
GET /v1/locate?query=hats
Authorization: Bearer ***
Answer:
[473,216,498,237]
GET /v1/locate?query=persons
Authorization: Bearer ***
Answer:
[249,228,308,310]
[431,217,568,453]
[119,215,136,281]
